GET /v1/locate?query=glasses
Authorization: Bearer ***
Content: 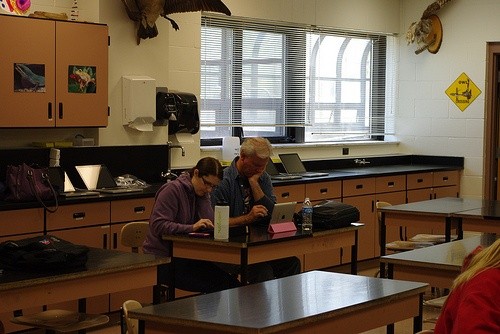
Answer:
[201,176,217,189]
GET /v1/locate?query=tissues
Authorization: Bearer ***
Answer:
[121,73,156,133]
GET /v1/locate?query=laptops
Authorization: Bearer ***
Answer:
[265,157,302,180]
[75,164,144,193]
[278,153,329,177]
[49,169,98,196]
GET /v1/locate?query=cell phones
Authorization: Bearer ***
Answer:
[188,232,210,239]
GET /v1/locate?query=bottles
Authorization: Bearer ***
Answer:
[301,197,312,230]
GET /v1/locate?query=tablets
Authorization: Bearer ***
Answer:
[269,202,297,224]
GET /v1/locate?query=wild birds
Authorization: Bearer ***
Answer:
[121,0,231,46]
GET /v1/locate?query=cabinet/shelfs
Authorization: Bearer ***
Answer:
[0,160,499,333]
[0,14,111,128]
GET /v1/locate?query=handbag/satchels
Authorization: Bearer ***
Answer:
[7,162,59,213]
[0,234,90,274]
[294,199,360,230]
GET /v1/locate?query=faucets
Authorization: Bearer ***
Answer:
[167,140,186,183]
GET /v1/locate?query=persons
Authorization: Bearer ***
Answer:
[211,137,279,289]
[432,236,500,333]
[145,159,241,292]
